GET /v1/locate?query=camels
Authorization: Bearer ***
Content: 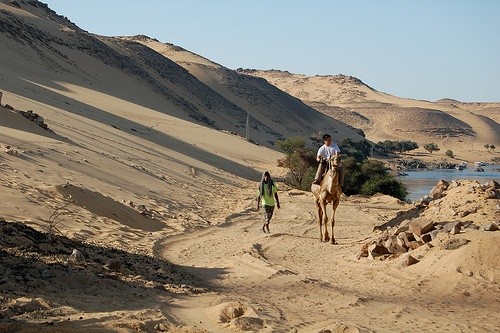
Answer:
[311,154,342,245]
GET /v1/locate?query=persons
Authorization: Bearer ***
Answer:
[255,171,280,233]
[312,133,345,188]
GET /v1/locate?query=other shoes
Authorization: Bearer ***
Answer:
[312,180,320,184]
